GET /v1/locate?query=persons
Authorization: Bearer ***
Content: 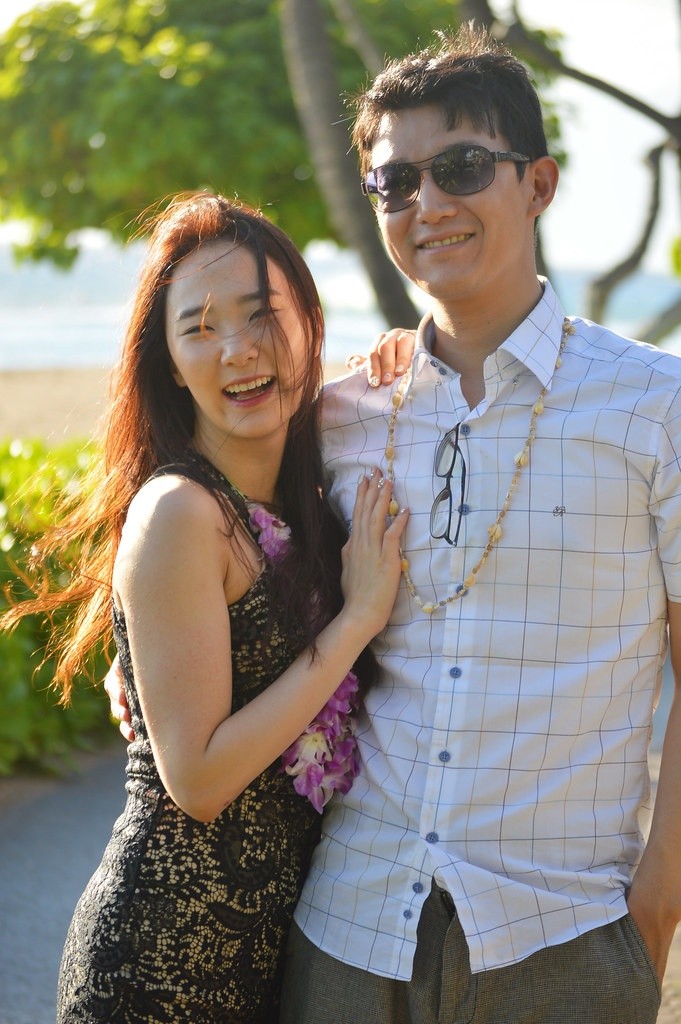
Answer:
[103,53,681,1024]
[55,192,417,1024]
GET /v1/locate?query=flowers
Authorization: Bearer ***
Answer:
[244,495,365,815]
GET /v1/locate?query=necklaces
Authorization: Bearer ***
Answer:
[385,317,576,615]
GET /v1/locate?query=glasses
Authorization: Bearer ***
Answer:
[361,145,531,213]
[430,422,466,547]
